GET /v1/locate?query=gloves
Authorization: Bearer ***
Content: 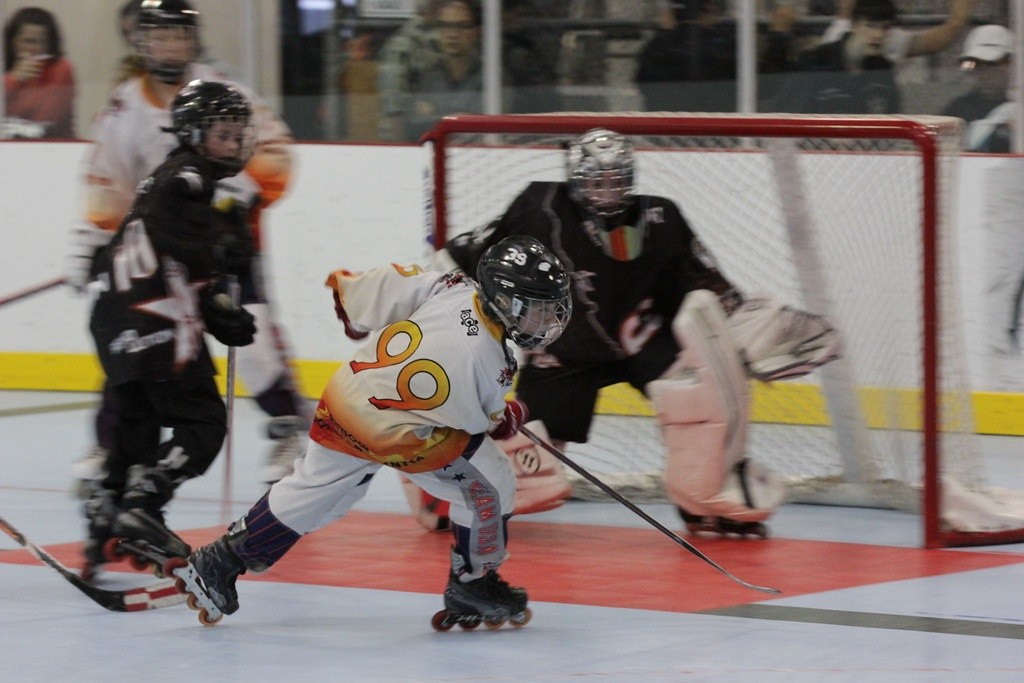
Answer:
[211,171,261,214]
[195,284,258,348]
[490,399,528,440]
[63,219,115,292]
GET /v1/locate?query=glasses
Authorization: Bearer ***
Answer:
[435,20,480,31]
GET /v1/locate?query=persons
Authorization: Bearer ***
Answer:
[67,0,315,499]
[377,1,1020,154]
[80,80,256,569]
[404,129,843,535]
[164,237,570,632]
[112,0,143,87]
[4,8,76,139]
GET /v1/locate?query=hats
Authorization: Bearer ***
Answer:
[955,23,1017,67]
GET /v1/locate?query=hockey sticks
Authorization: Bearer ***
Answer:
[220,272,244,524]
[518,425,785,596]
[0,276,67,306]
[0,515,191,614]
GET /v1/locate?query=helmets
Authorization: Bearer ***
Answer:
[476,235,572,351]
[118,0,205,85]
[169,80,256,179]
[564,127,638,217]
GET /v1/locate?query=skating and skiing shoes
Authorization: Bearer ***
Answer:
[431,544,533,633]
[162,514,269,626]
[104,462,193,578]
[259,393,314,486]
[671,456,781,540]
[80,486,121,582]
[72,446,110,501]
